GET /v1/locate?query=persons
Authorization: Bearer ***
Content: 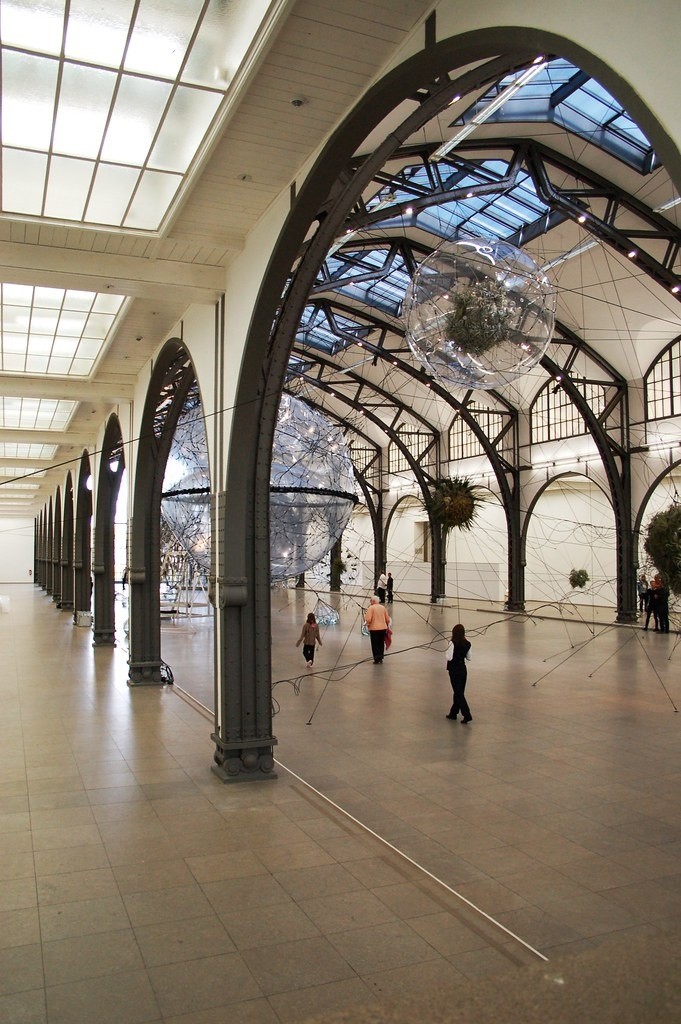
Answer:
[387,573,393,603]
[365,596,389,664]
[445,623,473,723]
[638,575,648,612]
[642,580,670,634]
[295,613,322,668]
[376,570,388,604]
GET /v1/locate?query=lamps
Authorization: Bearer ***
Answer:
[653,194,681,213]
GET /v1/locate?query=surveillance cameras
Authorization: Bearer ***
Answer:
[290,96,306,107]
[90,410,96,413]
[135,336,143,341]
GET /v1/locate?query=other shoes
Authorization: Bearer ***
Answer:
[653,628,658,631]
[306,660,313,668]
[446,714,457,720]
[373,661,379,664]
[461,714,472,723]
[642,627,647,631]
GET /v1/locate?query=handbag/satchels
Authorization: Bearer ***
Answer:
[384,626,392,650]
